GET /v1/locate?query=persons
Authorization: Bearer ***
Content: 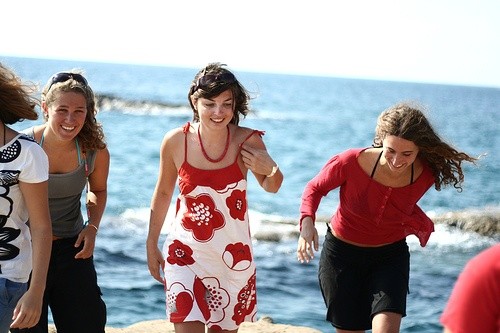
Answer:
[297,104,486,332]
[0,62,109,333]
[146,64,284,333]
[442,244,500,333]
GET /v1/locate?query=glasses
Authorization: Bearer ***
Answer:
[192,73,237,94]
[45,73,88,96]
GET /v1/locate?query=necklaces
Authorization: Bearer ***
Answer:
[198,124,230,163]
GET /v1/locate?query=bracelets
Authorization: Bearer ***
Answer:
[267,164,279,178]
[88,224,97,230]
